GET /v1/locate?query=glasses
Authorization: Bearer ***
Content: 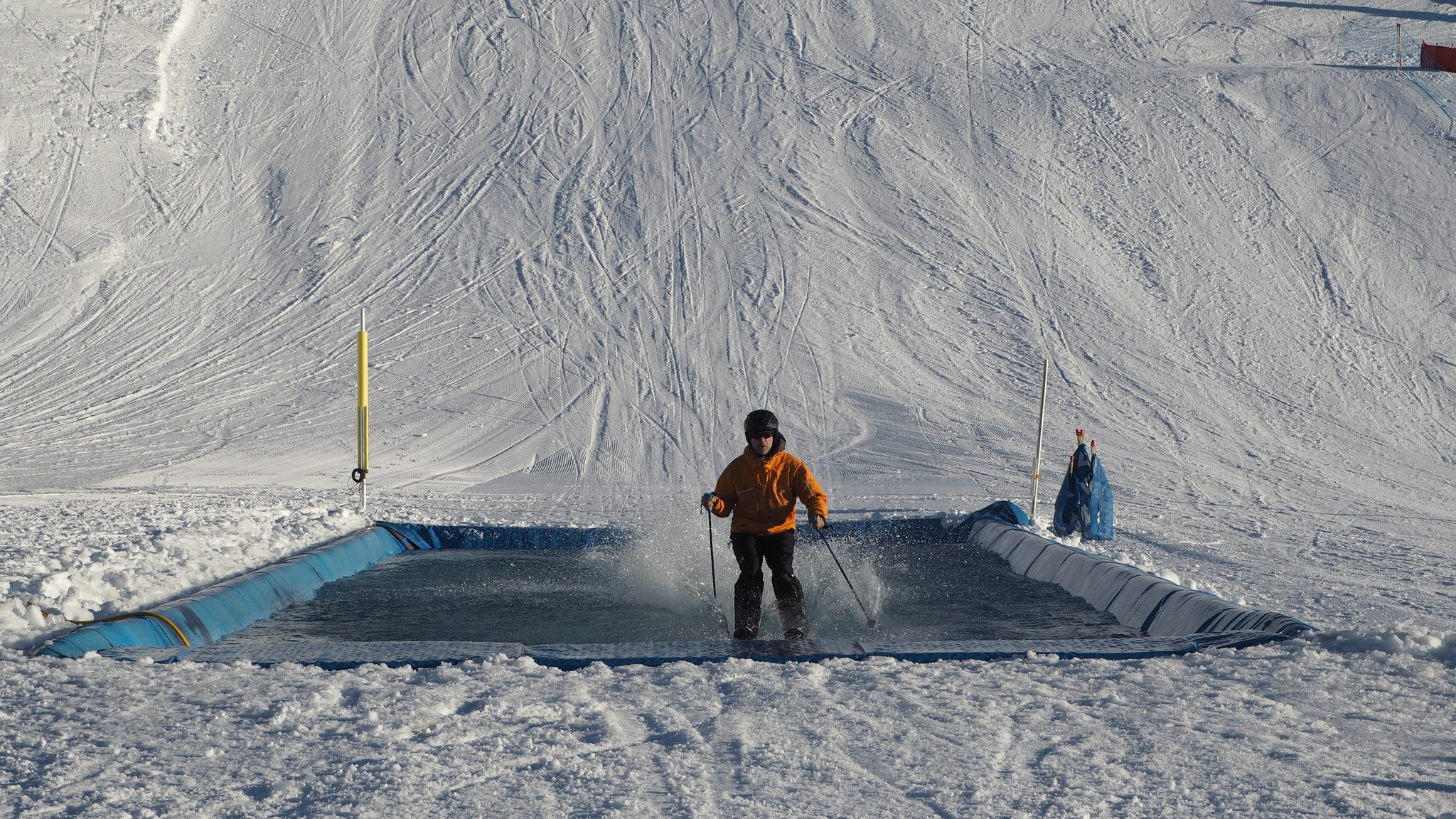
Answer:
[749,430,773,439]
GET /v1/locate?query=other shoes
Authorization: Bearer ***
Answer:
[784,626,809,638]
[733,626,757,640]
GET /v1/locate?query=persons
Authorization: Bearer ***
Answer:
[701,410,828,640]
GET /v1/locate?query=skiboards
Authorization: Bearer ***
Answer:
[726,631,813,643]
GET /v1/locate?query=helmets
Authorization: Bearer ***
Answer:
[744,410,779,436]
